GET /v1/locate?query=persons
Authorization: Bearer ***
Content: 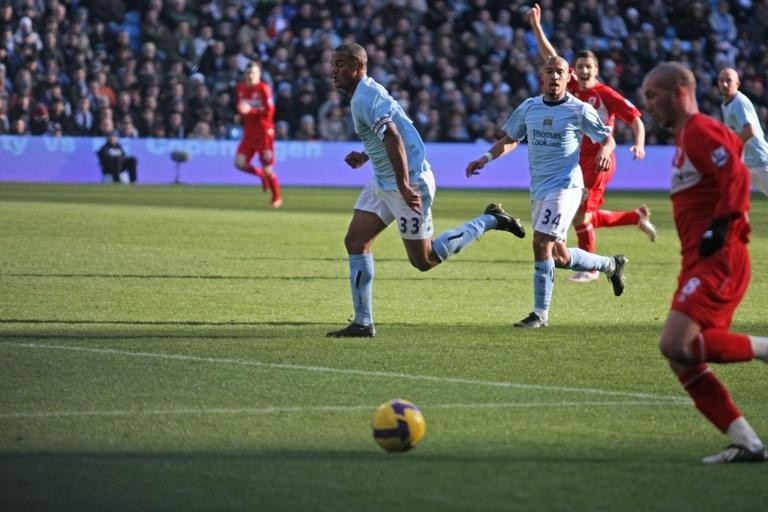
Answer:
[1,0,768,146]
[325,42,526,339]
[641,54,768,465]
[464,54,626,330]
[97,133,138,184]
[231,61,283,209]
[715,66,768,196]
[525,2,657,286]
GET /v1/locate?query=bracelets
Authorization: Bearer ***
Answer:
[483,150,493,163]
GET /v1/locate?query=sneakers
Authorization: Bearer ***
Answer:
[482,201,527,239]
[325,320,376,338]
[512,310,551,330]
[269,197,284,209]
[634,203,658,243]
[564,268,600,284]
[612,253,628,298]
[700,441,768,466]
[261,175,271,193]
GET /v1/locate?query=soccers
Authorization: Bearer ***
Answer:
[372,398,427,453]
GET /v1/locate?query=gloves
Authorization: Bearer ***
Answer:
[698,214,732,260]
